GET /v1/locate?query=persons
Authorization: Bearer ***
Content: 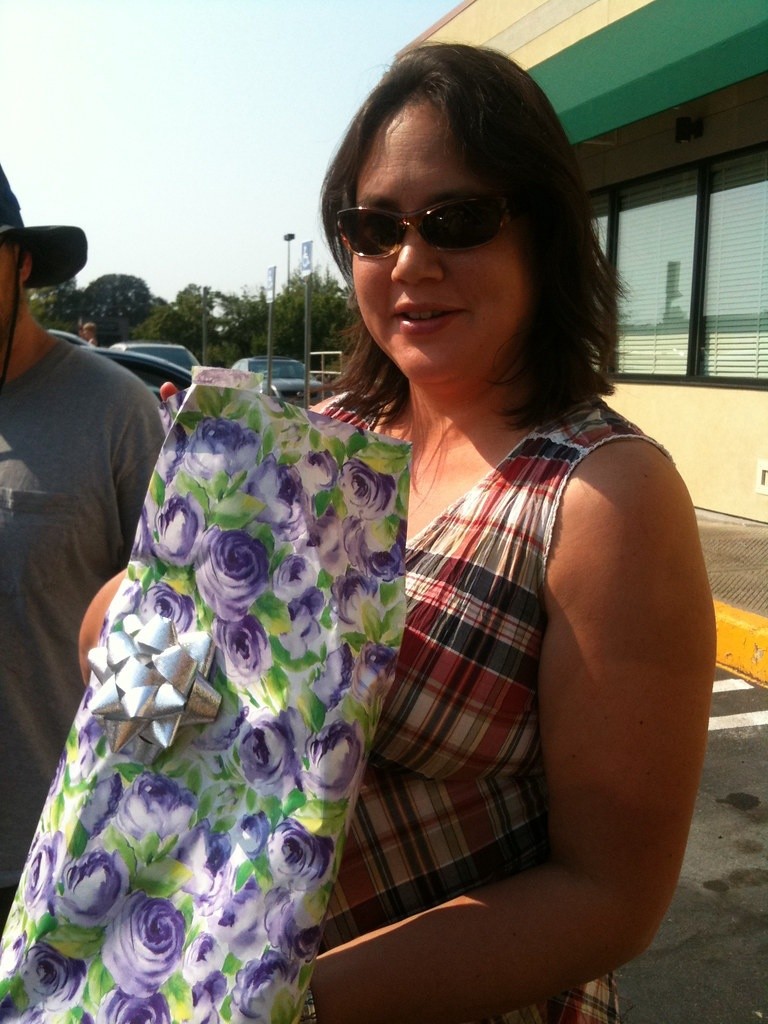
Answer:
[0,167,170,934]
[83,321,98,345]
[79,41,718,1023]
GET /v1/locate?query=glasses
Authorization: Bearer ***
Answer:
[333,190,536,256]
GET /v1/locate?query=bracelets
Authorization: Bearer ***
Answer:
[303,985,318,1022]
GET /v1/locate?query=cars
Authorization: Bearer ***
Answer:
[232,355,331,410]
[109,342,200,375]
[73,345,195,402]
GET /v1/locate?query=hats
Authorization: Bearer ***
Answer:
[0,164,89,290]
